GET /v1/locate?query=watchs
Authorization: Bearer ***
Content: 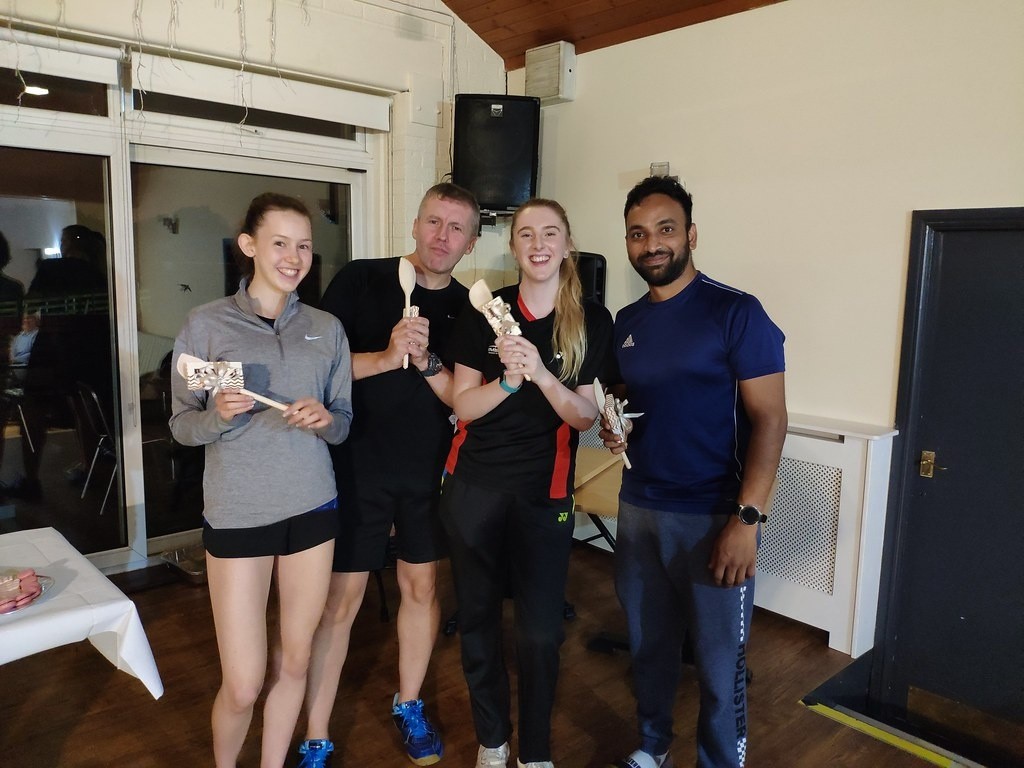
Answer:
[417,352,443,377]
[733,503,767,526]
[499,369,523,394]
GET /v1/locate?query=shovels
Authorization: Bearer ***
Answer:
[177,352,301,415]
[593,377,633,470]
[398,256,416,370]
[468,279,533,381]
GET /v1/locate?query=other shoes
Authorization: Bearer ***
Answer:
[70,473,102,487]
[621,748,671,768]
[8,473,38,500]
[0,480,9,496]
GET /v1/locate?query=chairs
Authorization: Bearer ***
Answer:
[79,350,173,515]
[0,292,108,332]
[0,322,96,453]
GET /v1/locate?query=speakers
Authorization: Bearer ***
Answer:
[451,94,542,216]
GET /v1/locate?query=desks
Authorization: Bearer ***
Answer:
[0,526,165,701]
[572,445,623,551]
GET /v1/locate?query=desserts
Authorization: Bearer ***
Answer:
[0,569,41,613]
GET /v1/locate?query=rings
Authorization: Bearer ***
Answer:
[424,344,429,348]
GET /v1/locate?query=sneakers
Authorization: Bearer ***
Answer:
[475,742,511,768]
[296,739,334,768]
[391,689,443,766]
[517,755,555,768]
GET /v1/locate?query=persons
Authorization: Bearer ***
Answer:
[437,198,616,768]
[296,184,480,768]
[5,312,39,388]
[168,192,353,768]
[0,224,108,502]
[597,175,787,768]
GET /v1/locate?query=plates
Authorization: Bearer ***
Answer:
[7,575,55,614]
